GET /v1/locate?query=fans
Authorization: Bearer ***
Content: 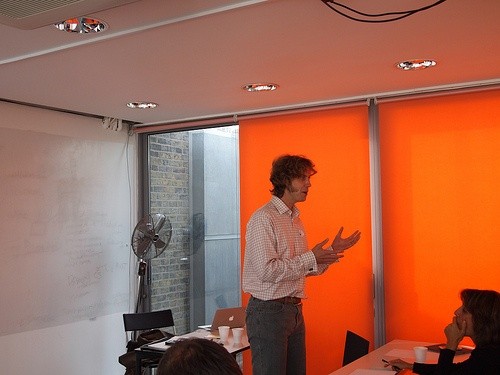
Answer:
[132,213,172,311]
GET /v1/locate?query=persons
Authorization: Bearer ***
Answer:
[242,155,361,375]
[156,337,243,375]
[384,289,500,375]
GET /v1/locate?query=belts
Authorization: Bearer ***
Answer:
[271,296,301,304]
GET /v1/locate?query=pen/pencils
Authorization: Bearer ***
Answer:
[382,359,400,373]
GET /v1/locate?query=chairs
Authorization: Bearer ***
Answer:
[343,331,369,366]
[123,309,176,346]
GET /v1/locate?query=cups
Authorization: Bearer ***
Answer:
[218,326,230,343]
[232,328,244,344]
[414,347,427,363]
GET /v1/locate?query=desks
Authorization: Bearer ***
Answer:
[133,327,250,375]
[330,339,474,375]
